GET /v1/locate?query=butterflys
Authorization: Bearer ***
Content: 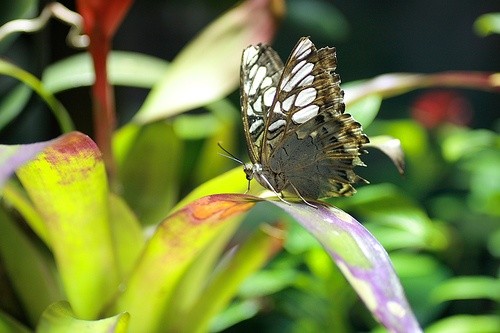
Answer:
[217,36,371,209]
